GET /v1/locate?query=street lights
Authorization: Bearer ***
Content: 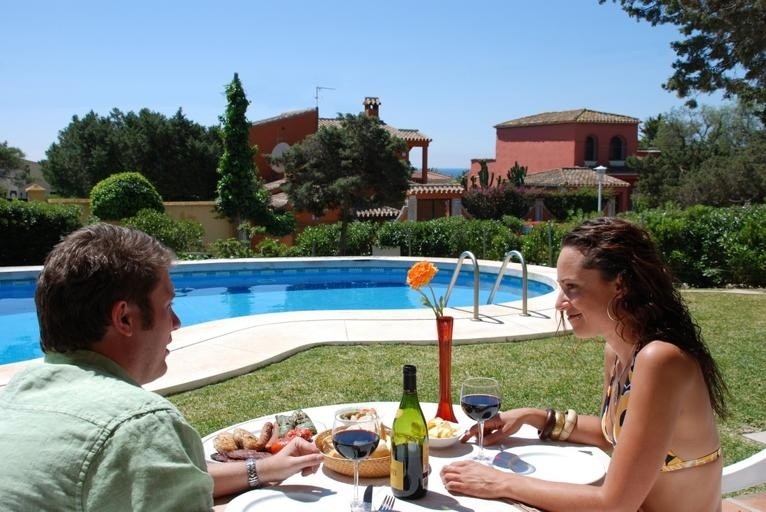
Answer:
[592,166,608,212]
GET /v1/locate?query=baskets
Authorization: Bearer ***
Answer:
[315,427,391,477]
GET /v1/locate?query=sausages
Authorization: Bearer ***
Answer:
[264,423,279,449]
[255,422,273,452]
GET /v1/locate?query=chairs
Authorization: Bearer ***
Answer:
[721,447,766,495]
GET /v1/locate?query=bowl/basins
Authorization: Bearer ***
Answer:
[428,432,465,449]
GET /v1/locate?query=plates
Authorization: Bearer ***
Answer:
[491,444,606,484]
[225,486,351,512]
[203,416,327,464]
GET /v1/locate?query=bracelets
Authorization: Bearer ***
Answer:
[245,457,260,491]
[550,411,565,440]
[560,408,577,440]
[538,407,556,440]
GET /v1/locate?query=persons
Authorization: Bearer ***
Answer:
[0,222,325,510]
[441,218,722,512]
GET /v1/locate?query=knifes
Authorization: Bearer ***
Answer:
[364,485,373,511]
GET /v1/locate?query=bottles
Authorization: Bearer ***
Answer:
[390,365,429,500]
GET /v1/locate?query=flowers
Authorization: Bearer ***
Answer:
[405,260,445,318]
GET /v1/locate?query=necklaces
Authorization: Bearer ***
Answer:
[612,342,639,409]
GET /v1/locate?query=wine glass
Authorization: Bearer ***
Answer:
[331,409,380,512]
[460,377,503,463]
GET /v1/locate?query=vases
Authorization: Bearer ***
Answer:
[434,317,459,424]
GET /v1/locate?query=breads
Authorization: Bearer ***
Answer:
[233,428,258,449]
[324,434,390,458]
[213,431,238,453]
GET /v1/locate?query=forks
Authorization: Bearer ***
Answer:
[377,496,395,512]
